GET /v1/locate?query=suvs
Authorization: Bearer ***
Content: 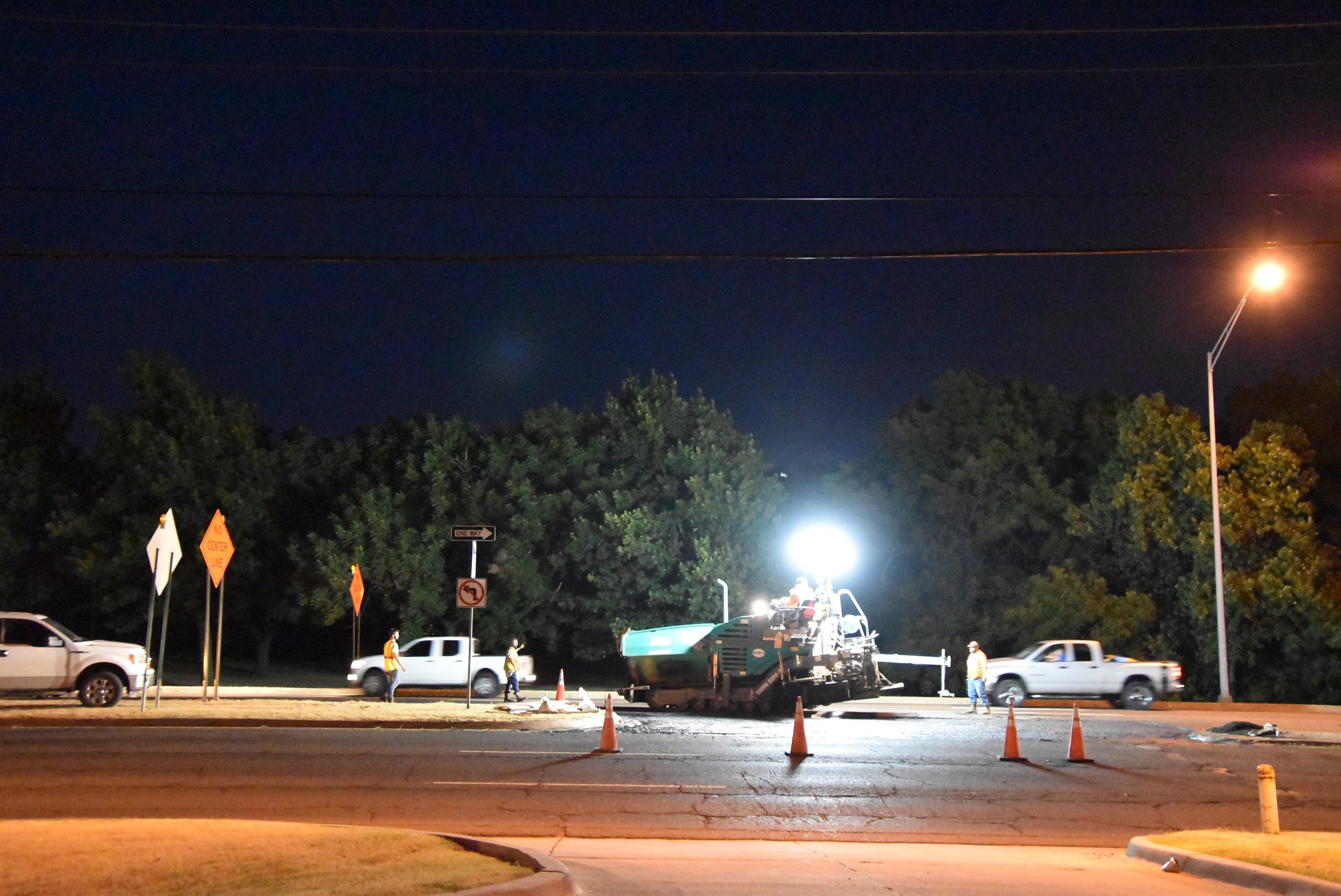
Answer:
[0,612,156,708]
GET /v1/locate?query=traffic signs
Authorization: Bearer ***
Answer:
[449,525,497,542]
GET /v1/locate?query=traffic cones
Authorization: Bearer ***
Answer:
[784,695,815,757]
[1060,702,1097,763]
[996,702,1030,762]
[594,693,624,754]
[555,668,566,703]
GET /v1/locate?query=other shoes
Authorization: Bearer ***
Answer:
[381,695,394,703]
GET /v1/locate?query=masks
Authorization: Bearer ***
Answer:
[969,648,976,652]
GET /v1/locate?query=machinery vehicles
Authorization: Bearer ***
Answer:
[619,581,901,715]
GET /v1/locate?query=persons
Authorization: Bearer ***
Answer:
[503,637,527,703]
[384,628,406,703]
[809,571,833,639]
[789,577,814,606]
[967,642,991,714]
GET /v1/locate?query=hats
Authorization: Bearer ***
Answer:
[967,641,979,648]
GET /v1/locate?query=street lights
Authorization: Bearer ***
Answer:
[1204,262,1290,698]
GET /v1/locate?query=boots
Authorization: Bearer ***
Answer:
[983,707,991,715]
[504,693,515,702]
[514,693,527,702]
[965,704,977,714]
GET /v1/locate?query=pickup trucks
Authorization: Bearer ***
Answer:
[348,636,538,700]
[983,639,1184,710]
